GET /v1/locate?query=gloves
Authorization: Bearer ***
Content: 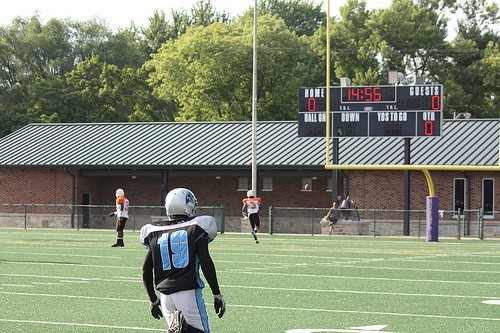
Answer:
[120,217,124,223]
[213,294,226,318]
[109,213,115,217]
[150,298,163,320]
[242,212,247,216]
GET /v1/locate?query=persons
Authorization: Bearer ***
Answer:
[324,202,342,235]
[109,189,129,247]
[242,190,262,244]
[140,187,227,333]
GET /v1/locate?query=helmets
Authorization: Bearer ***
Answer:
[115,189,124,198]
[246,190,255,198]
[165,188,198,218]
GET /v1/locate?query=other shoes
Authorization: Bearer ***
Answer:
[328,222,333,226]
[254,230,257,233]
[111,244,120,247]
[256,241,259,244]
[329,231,331,235]
